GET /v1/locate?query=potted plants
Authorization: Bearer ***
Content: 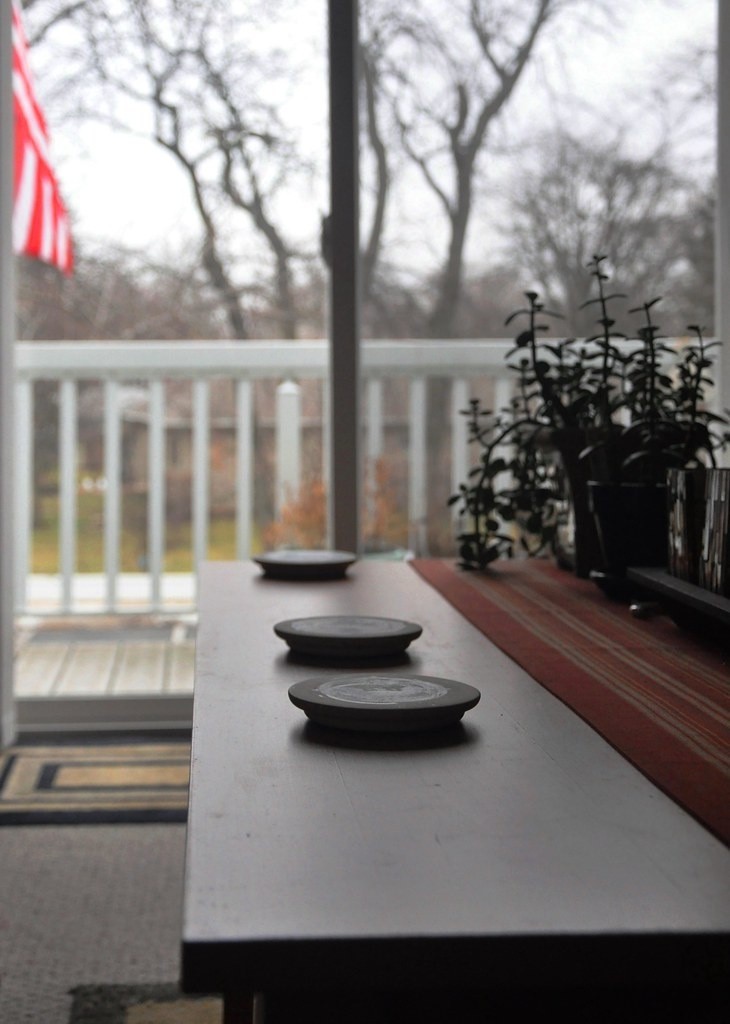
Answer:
[446,245,730,576]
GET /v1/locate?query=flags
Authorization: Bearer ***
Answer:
[12,0,74,276]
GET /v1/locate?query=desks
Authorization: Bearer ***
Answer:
[180,559,730,1024]
